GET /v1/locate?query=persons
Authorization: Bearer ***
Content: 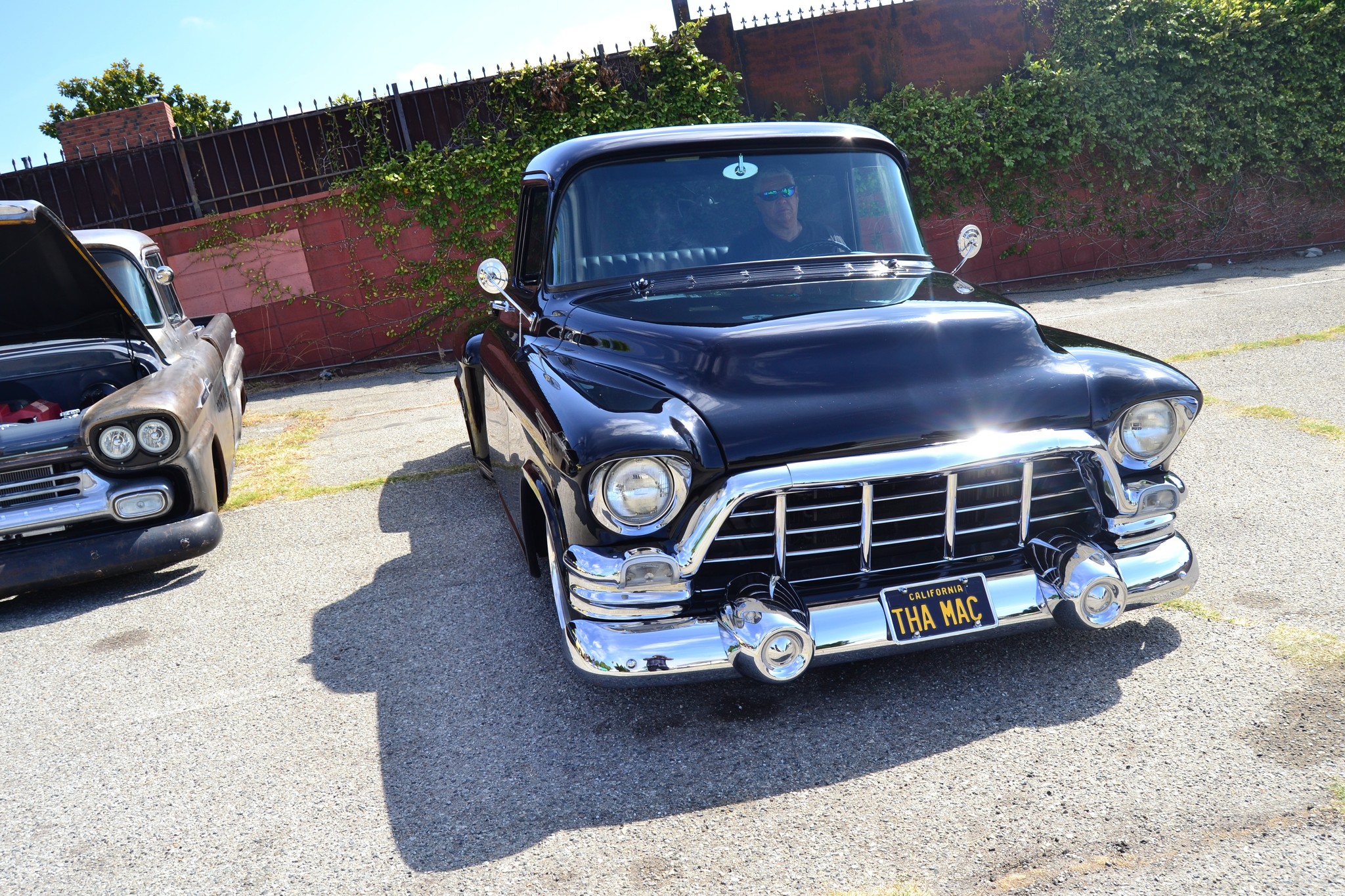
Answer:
[724,162,848,264]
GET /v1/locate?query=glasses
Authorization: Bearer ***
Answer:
[758,185,796,201]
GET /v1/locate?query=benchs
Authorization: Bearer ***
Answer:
[581,245,731,281]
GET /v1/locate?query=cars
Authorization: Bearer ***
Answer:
[453,119,1204,686]
[0,196,248,593]
